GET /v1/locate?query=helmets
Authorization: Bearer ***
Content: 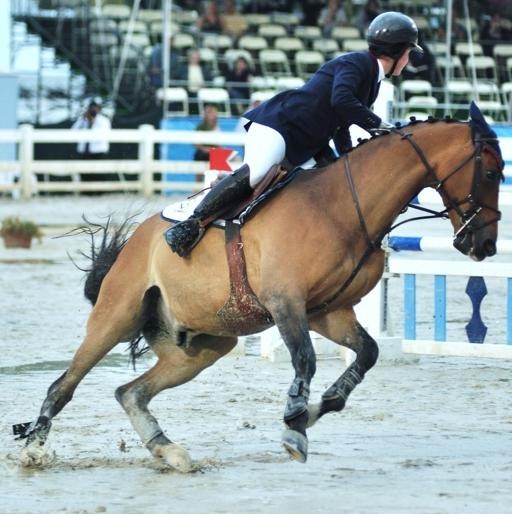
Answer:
[365,10,424,57]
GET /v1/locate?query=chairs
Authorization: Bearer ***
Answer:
[393,13,512,124]
[86,3,369,120]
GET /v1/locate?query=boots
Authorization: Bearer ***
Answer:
[161,160,256,259]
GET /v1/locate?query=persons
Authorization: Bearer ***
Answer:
[71,96,112,199]
[165,11,424,257]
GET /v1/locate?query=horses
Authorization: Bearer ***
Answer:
[23,99,506,474]
